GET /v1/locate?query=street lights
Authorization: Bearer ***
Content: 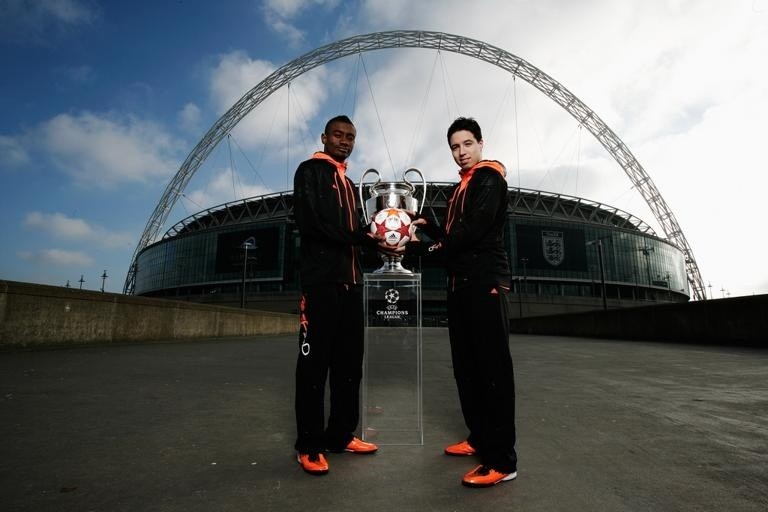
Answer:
[719,287,725,298]
[238,236,258,308]
[584,239,609,311]
[77,274,87,289]
[707,281,713,299]
[100,269,108,293]
[64,280,72,287]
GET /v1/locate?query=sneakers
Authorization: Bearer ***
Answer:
[344,436,378,454]
[444,440,477,455]
[298,451,328,475]
[462,463,517,487]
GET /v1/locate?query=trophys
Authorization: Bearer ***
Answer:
[359,167,427,278]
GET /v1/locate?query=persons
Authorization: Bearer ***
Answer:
[394,117,518,486]
[292,114,396,476]
